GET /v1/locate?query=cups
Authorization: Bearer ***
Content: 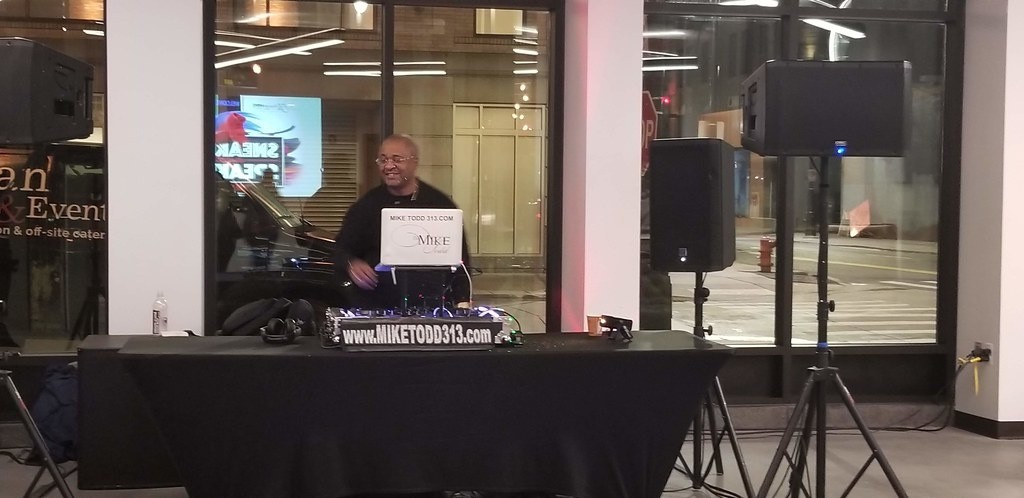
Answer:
[587,314,603,337]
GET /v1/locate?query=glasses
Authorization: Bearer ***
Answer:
[376,155,417,166]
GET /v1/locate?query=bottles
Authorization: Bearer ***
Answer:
[152,289,167,338]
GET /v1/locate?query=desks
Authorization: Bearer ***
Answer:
[120,330,730,498]
[76,335,182,489]
[22,339,79,465]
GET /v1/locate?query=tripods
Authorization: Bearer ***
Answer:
[670,272,755,498]
[756,157,909,498]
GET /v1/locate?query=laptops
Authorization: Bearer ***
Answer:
[375,207,463,272]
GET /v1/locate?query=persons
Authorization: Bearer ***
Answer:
[333,133,472,313]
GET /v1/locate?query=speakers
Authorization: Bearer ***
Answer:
[650,138,737,271]
[0,37,93,146]
[741,59,913,158]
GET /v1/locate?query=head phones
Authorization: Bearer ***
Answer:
[260,318,302,343]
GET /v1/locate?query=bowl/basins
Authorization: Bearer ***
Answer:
[261,326,302,345]
